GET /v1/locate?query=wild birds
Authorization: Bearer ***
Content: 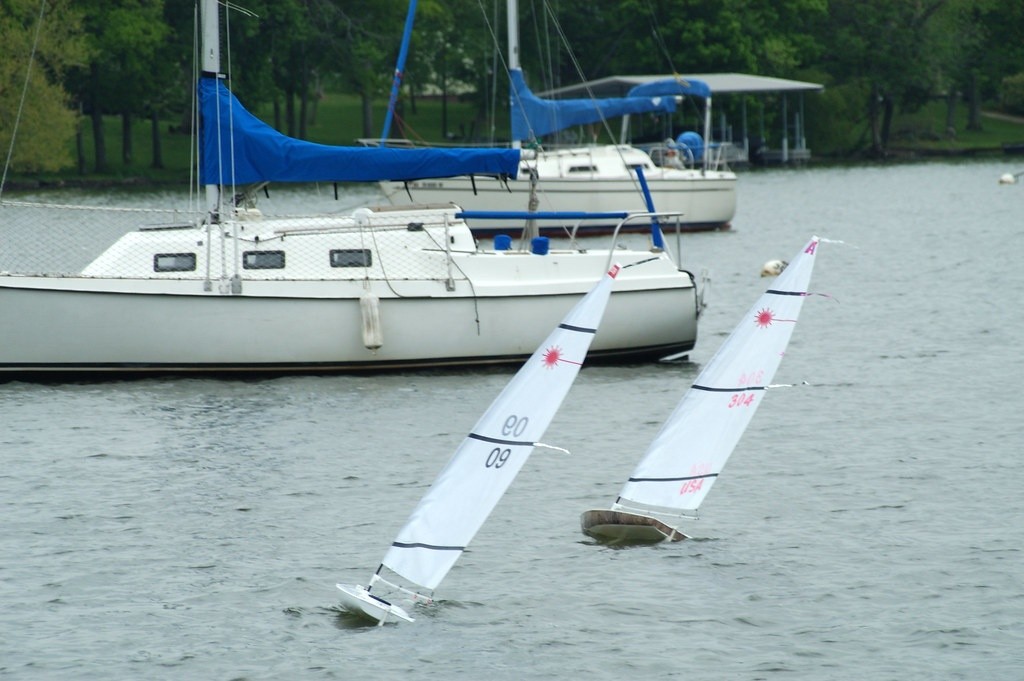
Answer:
[1000,171,1024,183]
[759,259,788,275]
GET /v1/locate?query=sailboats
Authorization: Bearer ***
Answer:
[350,0,738,242]
[580,232,823,548]
[337,265,620,627]
[1,0,702,359]
